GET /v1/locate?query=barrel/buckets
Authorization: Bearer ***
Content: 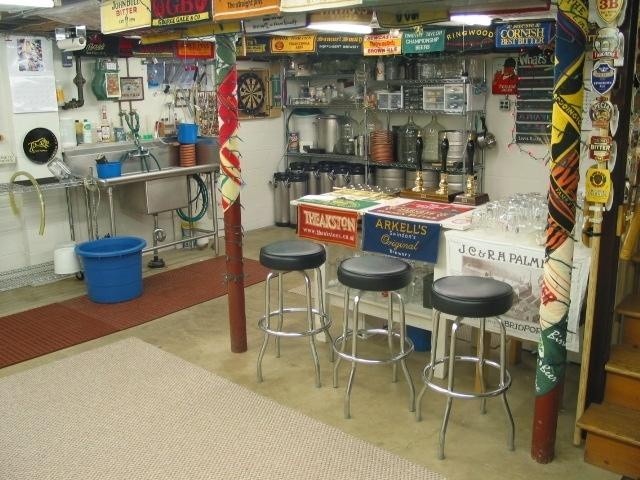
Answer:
[291,109,317,153]
[439,131,467,165]
[74,235,146,304]
[418,115,441,163]
[316,114,338,151]
[396,115,420,165]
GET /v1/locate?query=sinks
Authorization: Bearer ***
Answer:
[123,168,174,177]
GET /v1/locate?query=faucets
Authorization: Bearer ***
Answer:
[128,146,154,161]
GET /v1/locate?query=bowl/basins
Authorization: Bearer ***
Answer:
[181,145,196,167]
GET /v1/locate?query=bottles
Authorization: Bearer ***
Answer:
[74,119,92,144]
[100,106,113,144]
[375,57,386,80]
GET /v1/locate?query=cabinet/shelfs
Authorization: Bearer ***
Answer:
[286,59,486,206]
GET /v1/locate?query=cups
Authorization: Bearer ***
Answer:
[467,191,589,248]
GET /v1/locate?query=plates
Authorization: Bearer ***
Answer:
[416,60,467,78]
